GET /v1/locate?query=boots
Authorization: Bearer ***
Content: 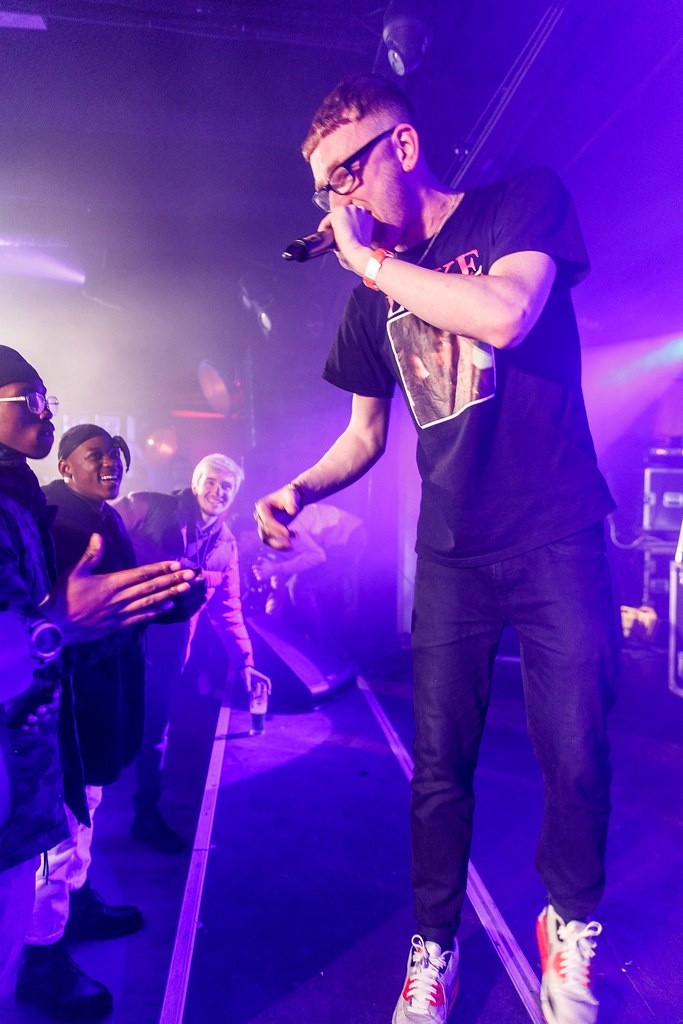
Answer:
[16,933,113,1023]
[67,875,142,942]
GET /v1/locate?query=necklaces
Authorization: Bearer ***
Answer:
[192,524,212,569]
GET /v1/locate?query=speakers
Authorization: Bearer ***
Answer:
[242,611,357,707]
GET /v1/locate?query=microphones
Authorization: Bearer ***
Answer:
[281,226,334,263]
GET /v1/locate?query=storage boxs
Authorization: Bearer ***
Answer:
[643,468,683,696]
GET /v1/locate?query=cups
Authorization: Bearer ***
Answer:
[248,691,267,735]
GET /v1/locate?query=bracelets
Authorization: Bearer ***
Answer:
[363,247,398,292]
[282,482,304,512]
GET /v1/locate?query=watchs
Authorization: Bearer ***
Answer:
[22,607,65,684]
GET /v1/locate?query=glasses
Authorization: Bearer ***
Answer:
[0,392,60,412]
[312,126,395,213]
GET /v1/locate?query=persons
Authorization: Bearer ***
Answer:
[253,74,617,1023]
[0,342,383,1024]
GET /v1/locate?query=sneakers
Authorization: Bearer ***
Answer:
[391,926,459,1024]
[535,899,602,1023]
[134,813,189,855]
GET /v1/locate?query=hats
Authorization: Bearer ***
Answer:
[0,344,46,386]
[56,425,114,459]
